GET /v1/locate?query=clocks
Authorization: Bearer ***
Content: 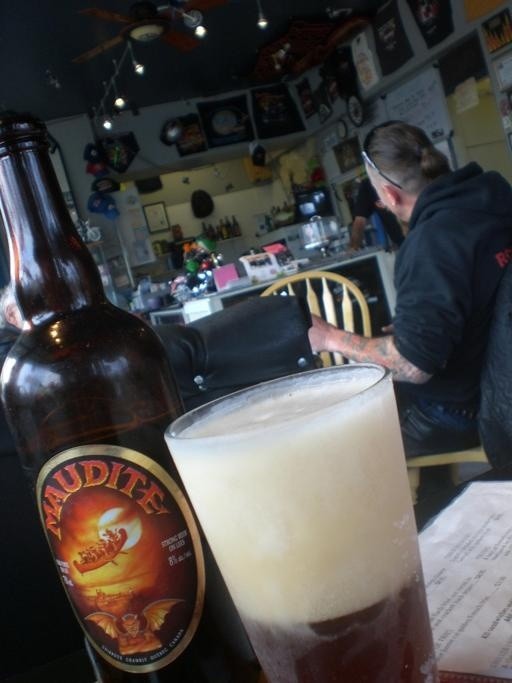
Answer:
[207,104,243,141]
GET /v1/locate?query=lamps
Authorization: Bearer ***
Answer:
[89,39,146,130]
[161,111,181,145]
[179,1,280,36]
[131,24,164,38]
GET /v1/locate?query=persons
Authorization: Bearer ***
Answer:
[116,613,164,656]
[306,117,512,463]
[346,175,406,253]
[0,280,23,376]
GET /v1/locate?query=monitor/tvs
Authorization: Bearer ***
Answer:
[298,201,316,216]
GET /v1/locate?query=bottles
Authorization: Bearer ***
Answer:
[0,113,258,683]
[201,214,242,240]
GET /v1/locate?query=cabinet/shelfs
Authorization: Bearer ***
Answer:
[80,220,136,310]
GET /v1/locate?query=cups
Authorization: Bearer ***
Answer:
[161,362,439,683]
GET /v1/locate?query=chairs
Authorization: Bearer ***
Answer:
[254,268,376,369]
[165,291,314,412]
[406,231,512,508]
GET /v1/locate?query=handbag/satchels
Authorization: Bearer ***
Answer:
[155,294,317,420]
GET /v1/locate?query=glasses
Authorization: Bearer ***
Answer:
[361,119,405,189]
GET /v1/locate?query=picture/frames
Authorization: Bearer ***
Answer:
[344,96,365,126]
[144,197,172,235]
[326,136,363,171]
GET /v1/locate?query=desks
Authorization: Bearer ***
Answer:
[407,460,511,680]
